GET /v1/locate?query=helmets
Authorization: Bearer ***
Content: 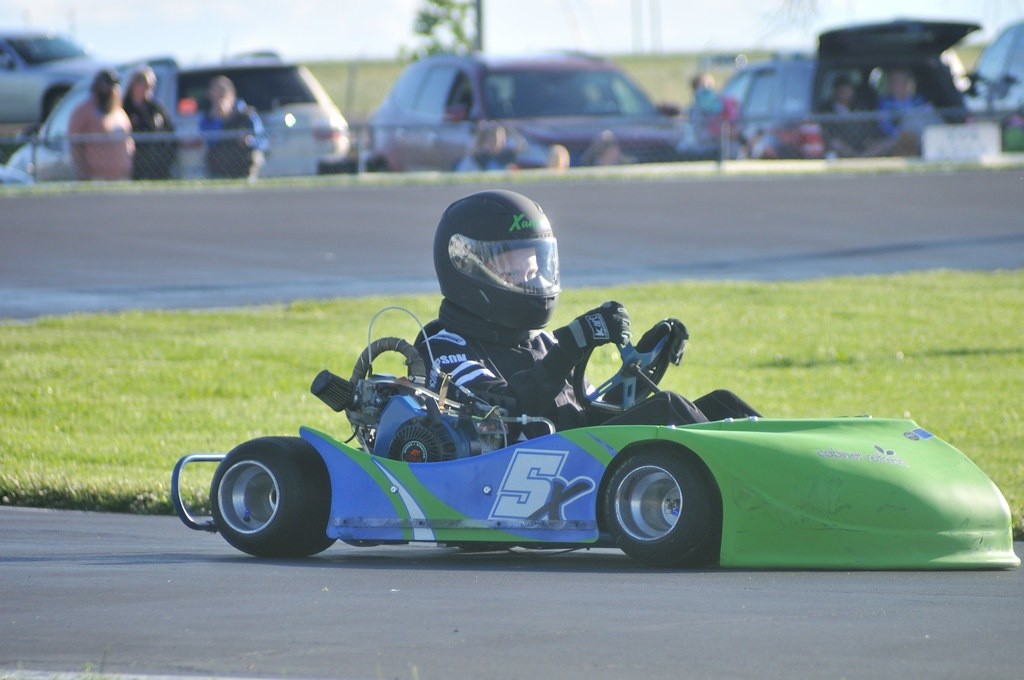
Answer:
[432,185,560,329]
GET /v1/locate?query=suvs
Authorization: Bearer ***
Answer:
[363,51,697,170]
[684,17,1024,159]
[8,50,355,181]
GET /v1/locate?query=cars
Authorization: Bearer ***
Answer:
[0,32,98,122]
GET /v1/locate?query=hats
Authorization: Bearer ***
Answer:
[95,69,120,82]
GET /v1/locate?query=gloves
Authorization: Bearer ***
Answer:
[634,317,689,366]
[553,299,634,361]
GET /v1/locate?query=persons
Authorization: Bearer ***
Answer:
[418,189,764,438]
[195,75,269,180]
[450,69,947,172]
[66,64,180,180]
